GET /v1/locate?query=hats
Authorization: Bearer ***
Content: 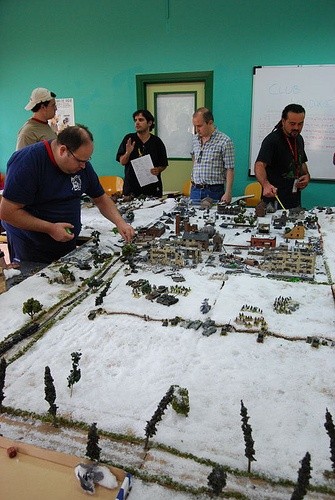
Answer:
[24,87,56,111]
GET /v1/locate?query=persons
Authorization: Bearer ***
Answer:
[16,88,59,149]
[190,107,234,203]
[254,104,310,212]
[0,124,135,276]
[116,110,168,199]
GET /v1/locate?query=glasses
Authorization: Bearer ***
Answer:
[62,143,91,163]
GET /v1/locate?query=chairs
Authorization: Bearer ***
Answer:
[245,181,263,207]
[98,176,124,195]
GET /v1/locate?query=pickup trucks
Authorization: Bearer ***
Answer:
[88,310,96,320]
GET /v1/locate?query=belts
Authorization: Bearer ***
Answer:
[191,182,215,189]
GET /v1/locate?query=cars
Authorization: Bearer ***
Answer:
[257,334,263,343]
[220,327,227,335]
[311,338,319,347]
